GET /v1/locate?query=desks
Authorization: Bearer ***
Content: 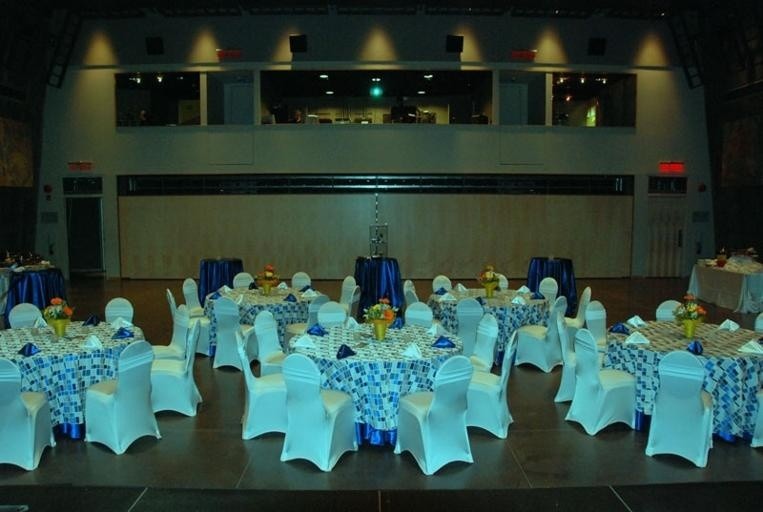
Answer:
[352,257,404,327]
[526,256,578,318]
[198,258,243,307]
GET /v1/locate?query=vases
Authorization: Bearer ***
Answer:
[682,319,698,339]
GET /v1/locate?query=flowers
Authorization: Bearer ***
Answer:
[673,291,706,320]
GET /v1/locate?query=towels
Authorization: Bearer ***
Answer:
[714,318,739,332]
[625,330,650,345]
[737,339,763,355]
[625,315,646,328]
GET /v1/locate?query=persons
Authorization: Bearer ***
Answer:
[286,108,305,123]
[137,109,153,126]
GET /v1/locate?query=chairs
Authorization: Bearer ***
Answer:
[654,298,687,322]
[643,351,716,470]
[755,312,763,330]
[586,301,607,366]
[565,329,635,436]
